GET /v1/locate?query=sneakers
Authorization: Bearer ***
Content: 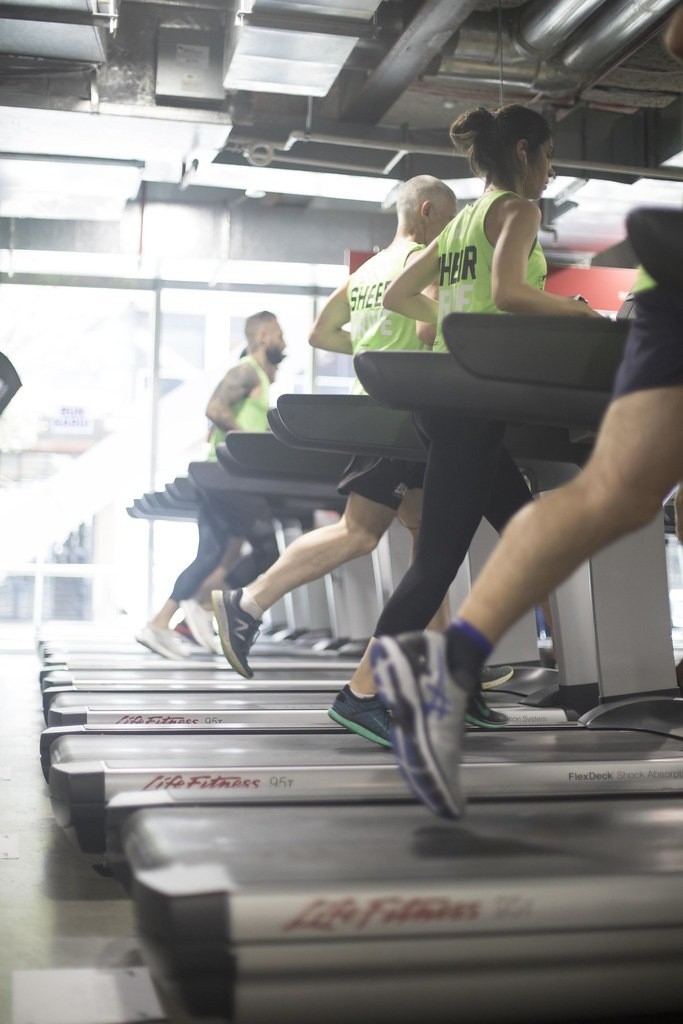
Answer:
[464,680,511,728]
[370,629,466,819]
[328,684,393,748]
[135,622,210,660]
[211,587,263,680]
[180,598,224,657]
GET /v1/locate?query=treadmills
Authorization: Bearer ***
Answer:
[31,203,683,1024]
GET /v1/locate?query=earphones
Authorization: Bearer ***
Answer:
[521,150,527,159]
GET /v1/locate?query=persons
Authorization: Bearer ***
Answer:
[209,175,514,690]
[367,260,681,822]
[134,312,286,657]
[328,106,608,747]
